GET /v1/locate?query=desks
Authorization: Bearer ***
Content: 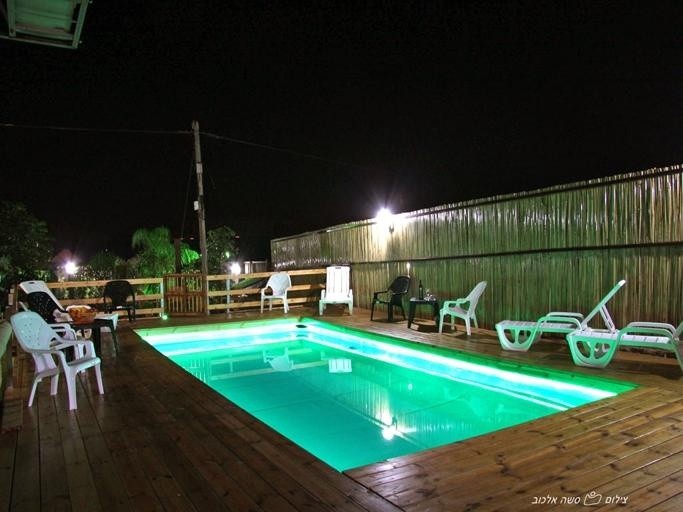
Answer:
[408,297,439,328]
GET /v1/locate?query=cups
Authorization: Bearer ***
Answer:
[425,288,430,299]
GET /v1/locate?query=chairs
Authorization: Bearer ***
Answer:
[261,272,292,315]
[370,276,410,322]
[565,321,683,371]
[495,280,626,351]
[0,280,134,410]
[319,265,353,316]
[439,280,487,336]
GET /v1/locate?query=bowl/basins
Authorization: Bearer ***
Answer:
[69,313,97,325]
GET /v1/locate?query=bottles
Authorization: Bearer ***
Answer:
[418,280,424,300]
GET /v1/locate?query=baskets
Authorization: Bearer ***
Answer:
[65,307,98,325]
